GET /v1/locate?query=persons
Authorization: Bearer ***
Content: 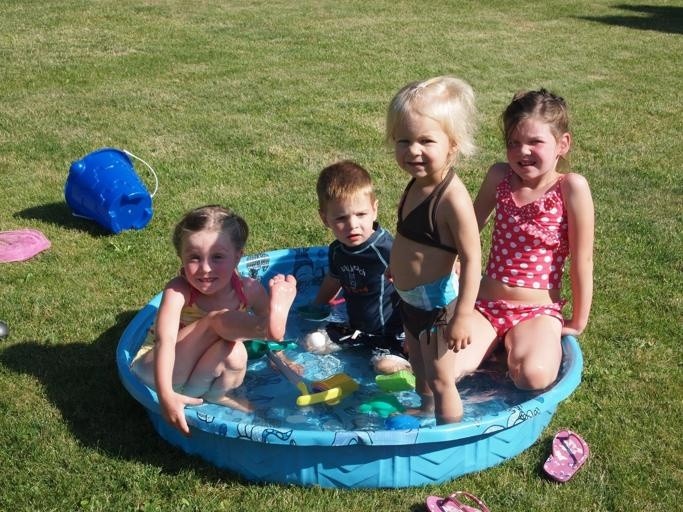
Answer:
[448,88,595,391]
[303,160,413,375]
[129,204,298,438]
[383,75,482,427]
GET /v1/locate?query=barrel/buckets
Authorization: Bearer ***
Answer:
[64,148,159,234]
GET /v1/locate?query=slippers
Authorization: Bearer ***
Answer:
[542,427,589,482]
[425,490,491,512]
